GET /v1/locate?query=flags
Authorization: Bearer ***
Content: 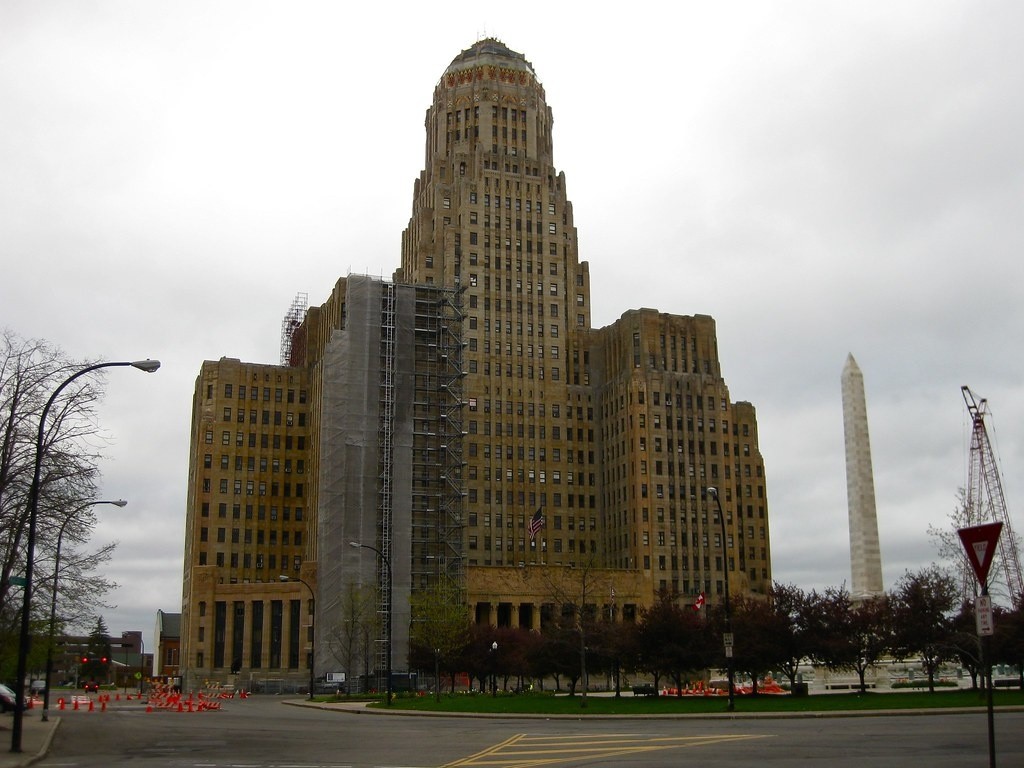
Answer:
[692,591,705,611]
[527,507,543,541]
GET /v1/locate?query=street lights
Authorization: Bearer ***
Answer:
[491,641,498,698]
[349,541,393,706]
[6,359,160,750]
[707,484,735,711]
[42,498,129,721]
[278,575,316,700]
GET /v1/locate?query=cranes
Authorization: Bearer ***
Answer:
[959,382,1024,611]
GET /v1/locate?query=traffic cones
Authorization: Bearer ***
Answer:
[127,695,134,700]
[98,695,103,702]
[101,701,107,712]
[188,690,248,712]
[145,700,153,712]
[88,699,95,712]
[116,693,120,700]
[59,699,66,710]
[73,700,80,710]
[106,694,110,702]
[176,702,184,712]
[28,697,35,709]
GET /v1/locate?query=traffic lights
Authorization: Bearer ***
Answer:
[102,657,107,663]
[82,657,88,663]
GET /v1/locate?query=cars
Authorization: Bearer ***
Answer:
[85,683,98,693]
[0,684,27,713]
